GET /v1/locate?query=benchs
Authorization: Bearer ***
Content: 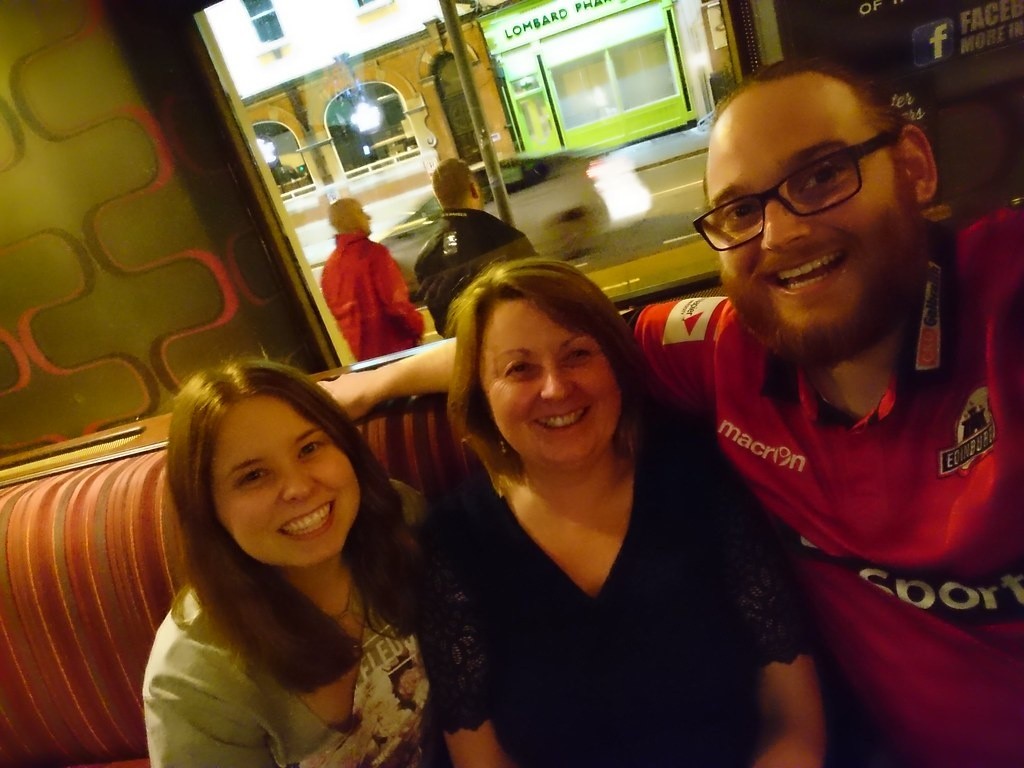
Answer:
[0,392,487,768]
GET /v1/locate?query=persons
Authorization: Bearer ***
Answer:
[142,356,430,768]
[318,53,1024,768]
[415,158,538,339]
[321,199,424,362]
[417,257,827,768]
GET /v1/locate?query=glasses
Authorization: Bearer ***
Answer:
[691,128,899,251]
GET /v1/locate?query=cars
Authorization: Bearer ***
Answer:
[368,148,652,303]
